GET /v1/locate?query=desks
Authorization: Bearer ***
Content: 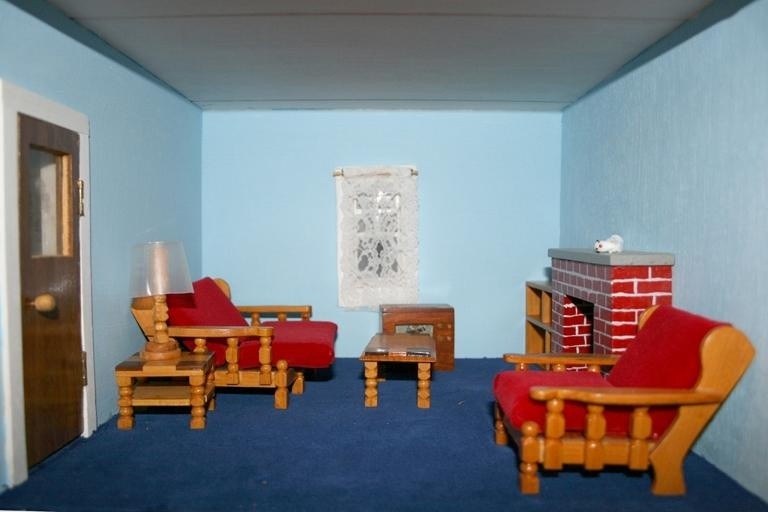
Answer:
[358,332,437,409]
[113,353,212,429]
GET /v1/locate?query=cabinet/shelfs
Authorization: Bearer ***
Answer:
[521,277,552,369]
[378,304,458,375]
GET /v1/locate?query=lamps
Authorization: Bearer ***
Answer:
[128,236,196,365]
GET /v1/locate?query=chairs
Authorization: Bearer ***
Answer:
[485,301,758,500]
[154,272,342,410]
